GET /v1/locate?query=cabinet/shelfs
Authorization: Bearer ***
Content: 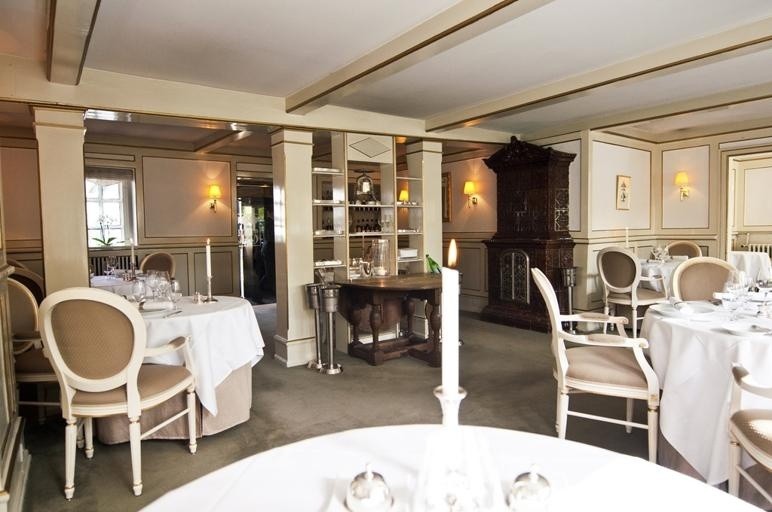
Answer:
[312,160,424,284]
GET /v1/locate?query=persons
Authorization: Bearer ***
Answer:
[251,207,276,305]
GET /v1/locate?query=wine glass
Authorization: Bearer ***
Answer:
[88,263,96,285]
[378,214,393,233]
[102,254,183,319]
[719,266,772,337]
[648,244,670,266]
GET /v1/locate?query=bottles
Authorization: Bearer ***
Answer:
[321,190,332,199]
[349,208,380,233]
[349,257,364,269]
[426,254,441,275]
[320,216,334,231]
[193,291,202,304]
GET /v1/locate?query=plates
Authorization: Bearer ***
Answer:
[649,301,717,320]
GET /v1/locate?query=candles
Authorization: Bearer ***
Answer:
[206,245,211,276]
[625,229,629,247]
[441,266,460,392]
[625,228,629,247]
[131,242,135,263]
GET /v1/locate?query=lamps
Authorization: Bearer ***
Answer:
[675,172,688,201]
[208,185,223,213]
[463,181,477,204]
[399,190,408,202]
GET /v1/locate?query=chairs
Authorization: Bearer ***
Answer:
[728,366,772,505]
[531,268,659,464]
[597,247,665,338]
[37,285,198,499]
[673,257,738,301]
[8,267,84,449]
[663,240,703,260]
[140,251,176,280]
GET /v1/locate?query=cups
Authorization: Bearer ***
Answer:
[333,223,343,235]
[372,238,391,276]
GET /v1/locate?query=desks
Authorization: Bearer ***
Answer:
[328,272,464,368]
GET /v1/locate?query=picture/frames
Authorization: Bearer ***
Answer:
[442,171,452,223]
[616,175,632,210]
